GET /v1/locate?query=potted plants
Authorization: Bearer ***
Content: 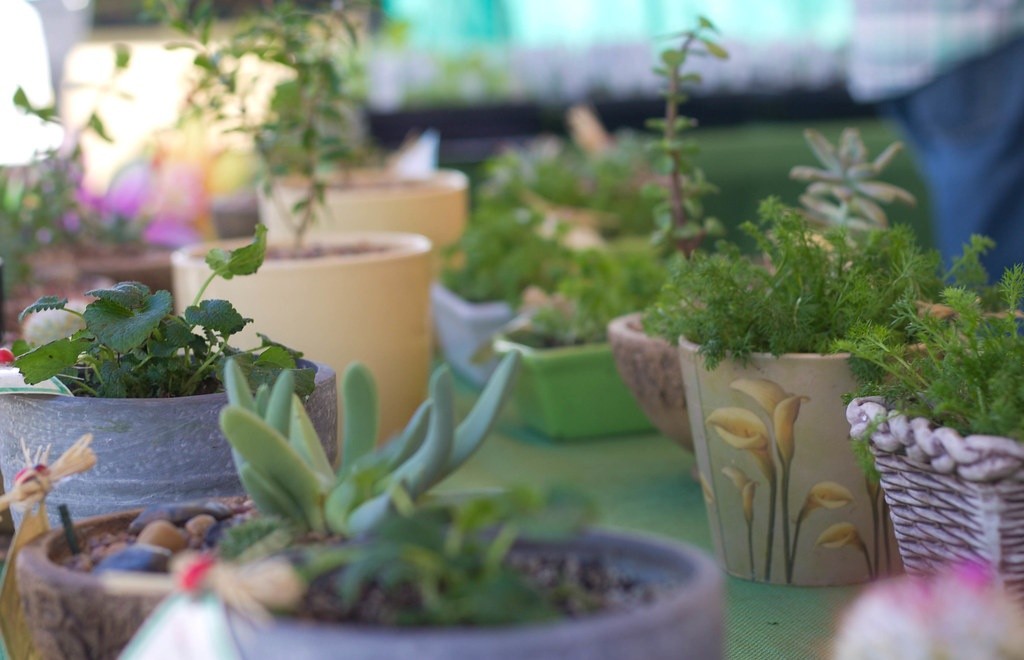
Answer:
[0,225,337,539]
[212,347,728,660]
[503,255,654,442]
[833,242,1024,598]
[642,200,911,590]
[430,170,575,384]
[605,266,693,452]
[65,0,433,468]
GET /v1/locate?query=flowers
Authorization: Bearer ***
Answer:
[71,161,217,255]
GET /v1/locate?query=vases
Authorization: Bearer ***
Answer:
[86,255,175,310]
[13,492,250,660]
[254,171,471,274]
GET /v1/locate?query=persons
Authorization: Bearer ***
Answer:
[846,1,1023,293]
